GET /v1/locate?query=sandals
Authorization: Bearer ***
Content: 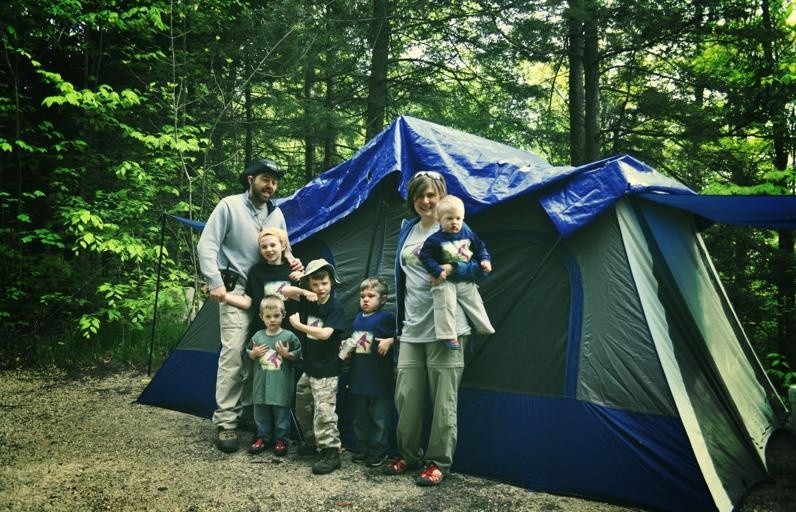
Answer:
[416,463,447,486]
[382,456,423,475]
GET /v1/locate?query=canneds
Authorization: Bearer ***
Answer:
[337,337,356,361]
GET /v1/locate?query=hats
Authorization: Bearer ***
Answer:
[300,258,335,284]
[240,159,287,190]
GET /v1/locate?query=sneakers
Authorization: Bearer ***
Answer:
[442,339,462,350]
[248,437,266,454]
[217,427,238,452]
[313,448,340,474]
[273,439,287,456]
[297,445,317,455]
[352,451,368,463]
[364,455,388,467]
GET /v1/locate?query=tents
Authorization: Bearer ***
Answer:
[137,116,795,512]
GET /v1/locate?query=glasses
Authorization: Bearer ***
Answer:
[407,171,441,185]
